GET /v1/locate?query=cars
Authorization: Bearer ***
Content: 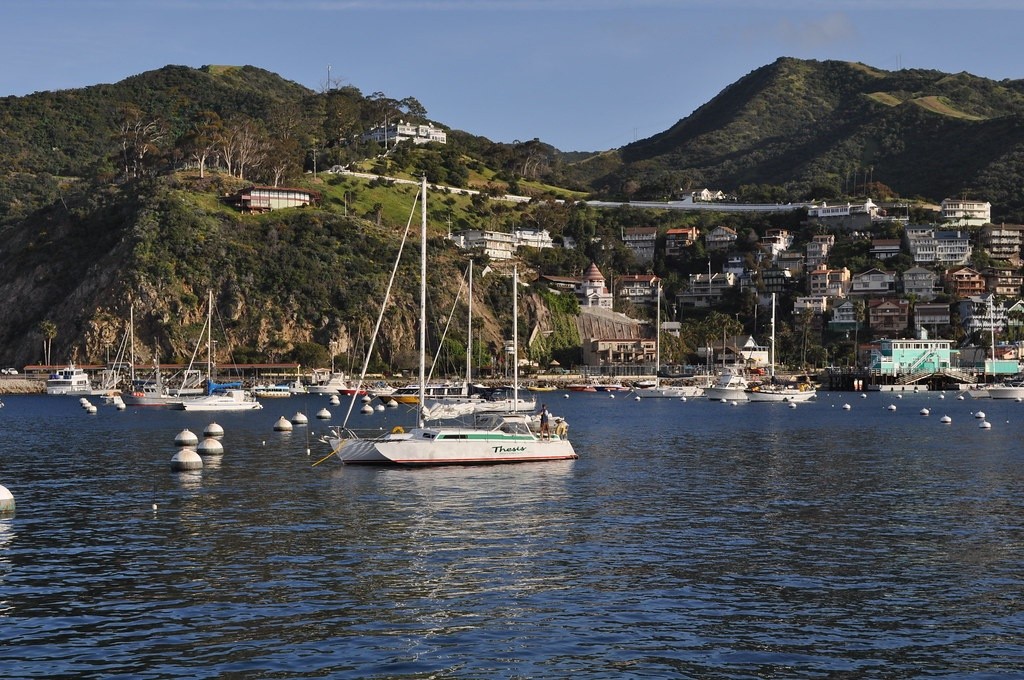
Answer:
[6,368,18,375]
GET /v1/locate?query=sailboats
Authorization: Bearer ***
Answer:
[323,177,578,465]
[165,291,264,410]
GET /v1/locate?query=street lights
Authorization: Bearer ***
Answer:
[980,292,996,384]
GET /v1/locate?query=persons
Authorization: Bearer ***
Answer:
[537,405,550,442]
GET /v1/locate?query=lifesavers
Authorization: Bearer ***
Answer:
[393,426,404,433]
[556,422,565,435]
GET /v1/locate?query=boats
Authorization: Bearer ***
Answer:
[744,293,816,401]
[47,360,92,395]
[308,373,348,393]
[987,380,1024,399]
[288,364,308,396]
[594,385,620,391]
[567,385,587,392]
[100,303,175,398]
[524,382,558,392]
[257,386,291,397]
[121,337,192,405]
[700,365,763,399]
[968,390,991,397]
[633,280,708,397]
